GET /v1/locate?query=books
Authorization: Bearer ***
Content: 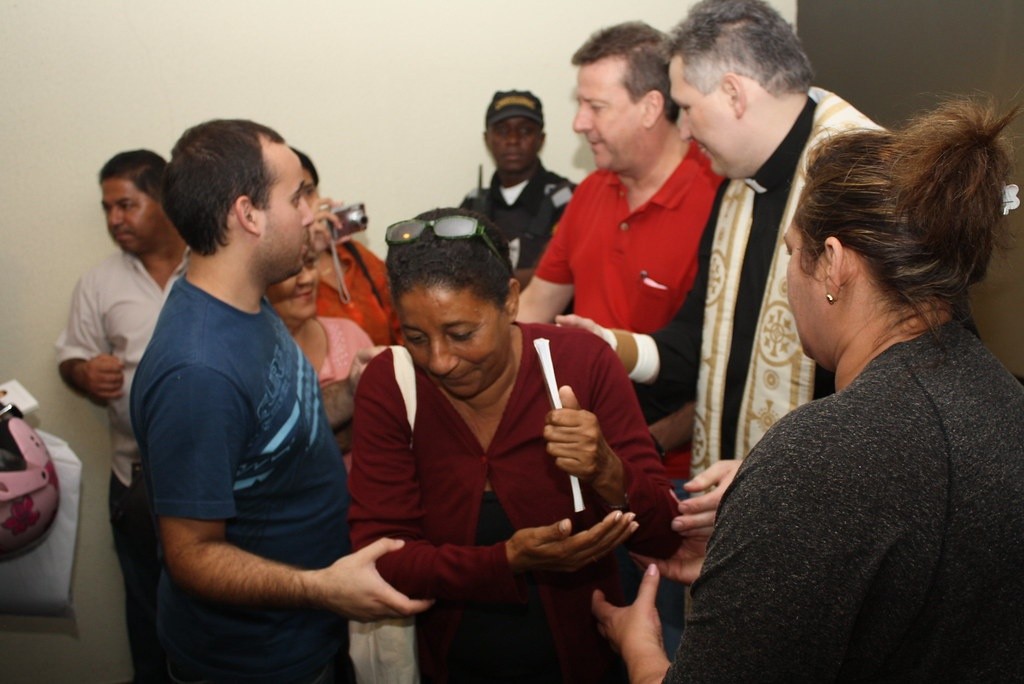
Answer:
[532,337,584,515]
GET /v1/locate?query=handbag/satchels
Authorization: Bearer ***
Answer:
[348,345,418,684]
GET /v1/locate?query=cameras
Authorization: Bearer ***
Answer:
[325,201,368,242]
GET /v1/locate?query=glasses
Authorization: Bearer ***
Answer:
[386,215,507,268]
[286,254,328,282]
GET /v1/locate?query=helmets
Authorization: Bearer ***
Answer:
[0,418,60,557]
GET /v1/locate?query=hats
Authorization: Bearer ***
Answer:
[485,90,545,132]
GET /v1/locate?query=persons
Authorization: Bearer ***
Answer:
[457,90,579,295]
[588,102,1024,684]
[263,253,376,478]
[56,149,191,684]
[287,143,407,349]
[349,208,686,684]
[126,117,435,683]
[509,21,730,666]
[553,1,897,626]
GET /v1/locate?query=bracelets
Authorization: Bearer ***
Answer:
[649,433,668,466]
